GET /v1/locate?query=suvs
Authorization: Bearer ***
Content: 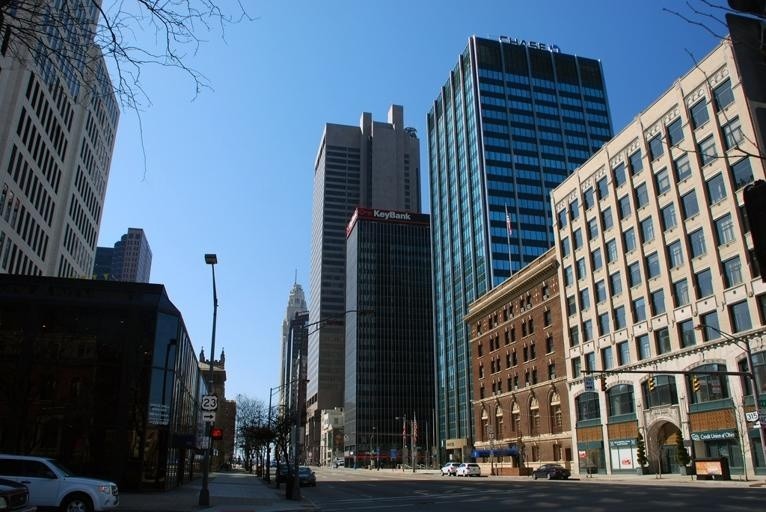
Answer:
[456,462,481,477]
[439,462,461,476]
[0,452,120,510]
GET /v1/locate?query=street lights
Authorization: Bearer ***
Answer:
[394,415,417,473]
[694,323,766,465]
[257,404,292,472]
[197,252,219,506]
[264,379,312,480]
[372,422,381,472]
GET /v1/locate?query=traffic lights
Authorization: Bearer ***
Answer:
[601,376,607,392]
[211,427,224,441]
[649,377,657,392]
[693,378,702,393]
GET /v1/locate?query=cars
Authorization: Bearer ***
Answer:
[531,463,572,480]
[274,464,318,488]
[580,454,599,476]
[0,479,38,512]
[257,460,280,467]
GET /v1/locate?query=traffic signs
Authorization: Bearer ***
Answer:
[585,378,594,390]
